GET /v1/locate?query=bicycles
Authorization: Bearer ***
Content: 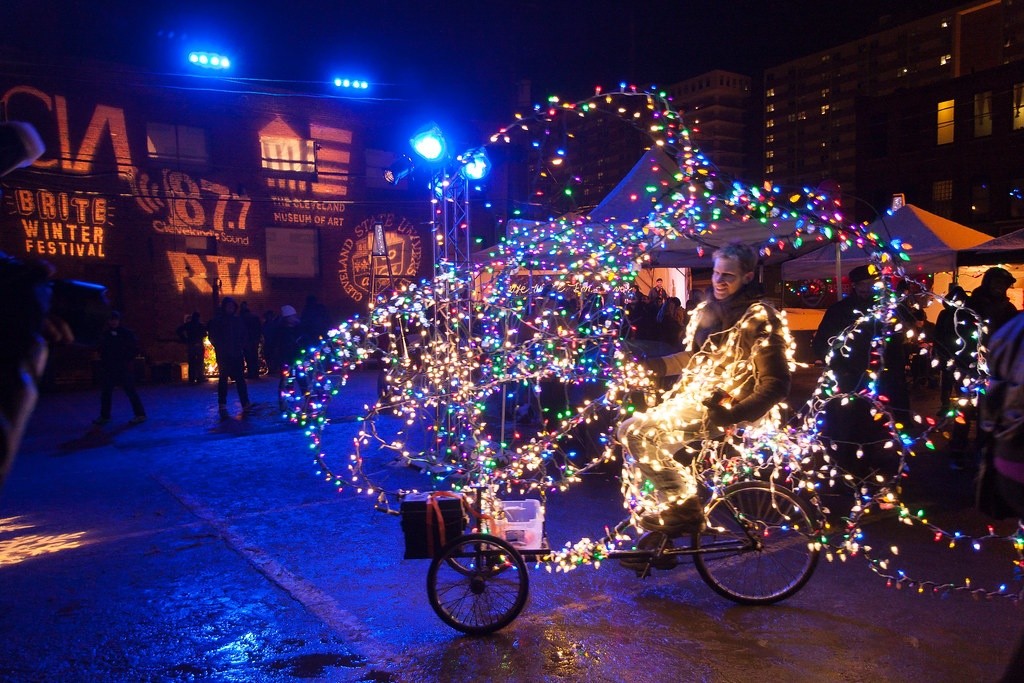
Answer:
[399,427,820,635]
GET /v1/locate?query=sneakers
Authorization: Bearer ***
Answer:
[640,496,705,532]
[618,533,679,567]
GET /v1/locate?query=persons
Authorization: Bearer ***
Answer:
[0,202,74,481]
[616,244,791,571]
[90,311,148,425]
[208,297,257,411]
[239,300,262,377]
[813,264,1024,521]
[176,312,210,383]
[271,305,312,412]
[261,310,280,375]
[630,277,695,349]
[300,294,331,343]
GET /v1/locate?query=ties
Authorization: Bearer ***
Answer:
[108,311,120,321]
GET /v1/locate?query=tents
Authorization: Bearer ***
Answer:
[952,228,1024,286]
[781,203,997,304]
[469,142,853,456]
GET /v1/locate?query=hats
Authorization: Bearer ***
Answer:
[221,297,234,306]
[981,267,1017,290]
[849,266,878,283]
[279,305,297,317]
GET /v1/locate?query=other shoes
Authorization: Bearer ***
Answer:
[947,439,964,452]
[91,416,111,423]
[130,415,146,425]
[937,406,949,415]
